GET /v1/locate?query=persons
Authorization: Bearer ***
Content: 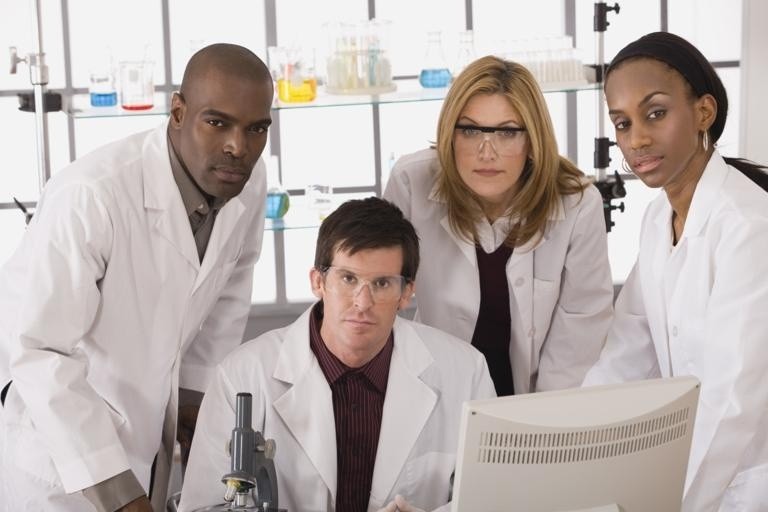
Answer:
[576,31,768,512]
[384,54,615,395]
[0,43,275,512]
[174,195,498,512]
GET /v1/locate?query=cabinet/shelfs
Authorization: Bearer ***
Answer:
[0,1,628,234]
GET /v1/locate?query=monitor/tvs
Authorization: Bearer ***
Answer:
[450,374,701,512]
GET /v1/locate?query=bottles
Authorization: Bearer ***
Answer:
[418,29,451,89]
[264,155,291,219]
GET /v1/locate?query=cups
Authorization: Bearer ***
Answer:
[88,72,118,107]
[268,43,318,104]
[118,60,160,111]
[303,182,334,213]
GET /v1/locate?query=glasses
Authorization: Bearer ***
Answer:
[317,265,410,304]
[449,124,528,155]
